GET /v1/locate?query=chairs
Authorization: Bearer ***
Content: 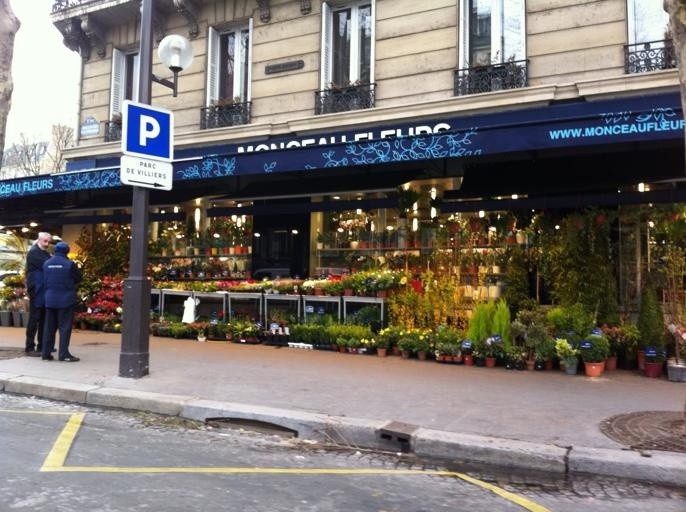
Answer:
[59,355,79,362]
[25,350,40,357]
[36,348,56,353]
[42,355,54,360]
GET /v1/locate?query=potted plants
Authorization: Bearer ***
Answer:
[0,185,686,381]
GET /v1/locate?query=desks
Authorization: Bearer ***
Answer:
[120,34,194,376]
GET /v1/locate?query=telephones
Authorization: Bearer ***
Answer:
[120,156,173,192]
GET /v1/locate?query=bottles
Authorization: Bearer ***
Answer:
[55,243,70,254]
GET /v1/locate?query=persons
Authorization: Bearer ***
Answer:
[25,231,58,358]
[41,241,81,363]
